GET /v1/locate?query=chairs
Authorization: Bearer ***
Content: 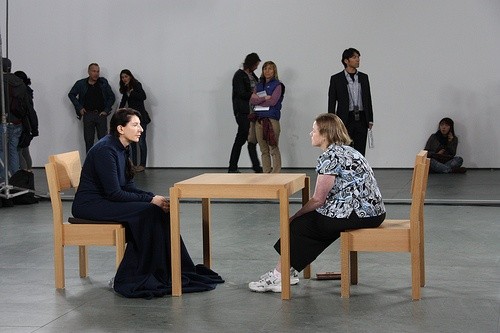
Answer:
[44,150,126,289]
[340,150,431,300]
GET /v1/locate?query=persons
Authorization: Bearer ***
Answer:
[71,109,224,300]
[228,53,264,173]
[248,114,387,292]
[327,48,373,157]
[0,57,32,177]
[14,70,39,174]
[119,69,152,172]
[249,61,286,173]
[68,63,116,152]
[425,117,467,173]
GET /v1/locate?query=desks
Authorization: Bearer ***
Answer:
[169,172,311,301]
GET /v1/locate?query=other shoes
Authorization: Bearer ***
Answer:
[452,167,466,173]
[136,166,144,172]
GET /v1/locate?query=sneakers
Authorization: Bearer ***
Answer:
[261,266,299,284]
[249,272,282,292]
